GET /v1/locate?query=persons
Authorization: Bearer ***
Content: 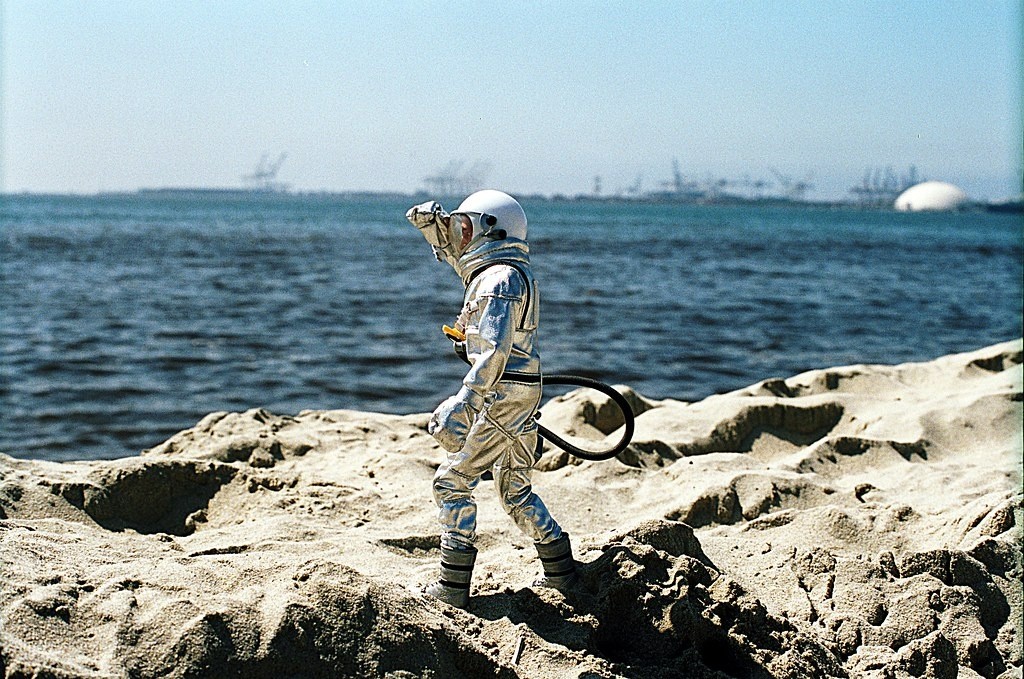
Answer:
[404,187,577,610]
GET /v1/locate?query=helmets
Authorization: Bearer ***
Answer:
[449,189,528,257]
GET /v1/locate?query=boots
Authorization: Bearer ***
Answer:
[534,533,574,585]
[430,550,478,607]
